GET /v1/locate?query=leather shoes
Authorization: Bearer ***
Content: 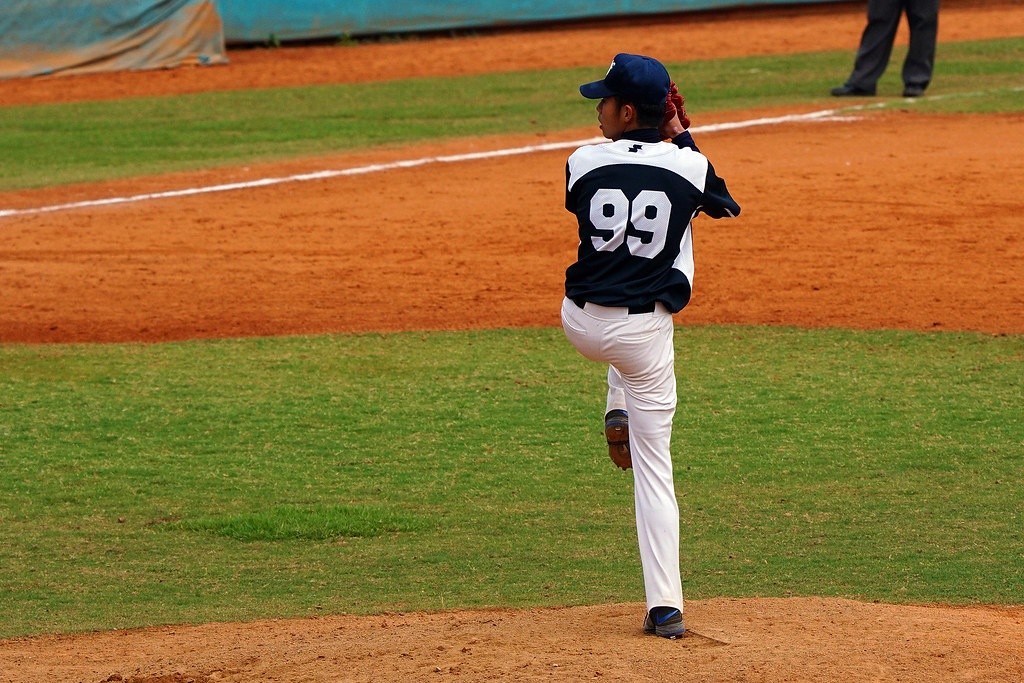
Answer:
[903,86,923,97]
[831,83,876,96]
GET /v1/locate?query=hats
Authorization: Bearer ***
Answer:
[579,52,671,99]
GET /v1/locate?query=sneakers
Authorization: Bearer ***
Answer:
[644,607,685,636]
[604,409,632,469]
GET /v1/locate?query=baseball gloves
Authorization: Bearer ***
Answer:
[665,80,694,128]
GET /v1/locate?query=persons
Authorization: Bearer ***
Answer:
[562,53,741,637]
[830,0,939,98]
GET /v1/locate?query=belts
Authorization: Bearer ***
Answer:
[572,298,656,314]
